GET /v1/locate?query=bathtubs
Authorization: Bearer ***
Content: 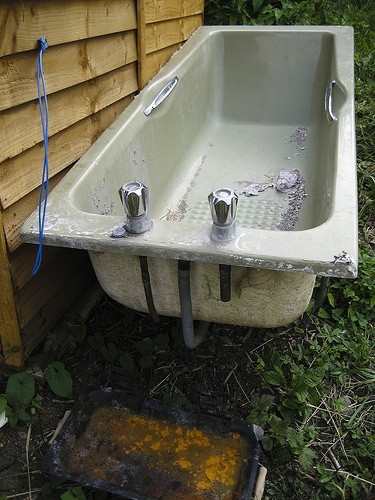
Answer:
[19,24,359,329]
